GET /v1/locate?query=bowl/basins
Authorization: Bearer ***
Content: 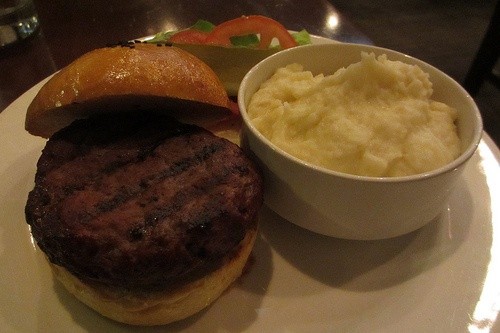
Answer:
[236,43,483,241]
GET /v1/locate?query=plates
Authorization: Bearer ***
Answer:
[0,30,500,332]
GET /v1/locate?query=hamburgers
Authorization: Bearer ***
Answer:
[23,37,264,325]
